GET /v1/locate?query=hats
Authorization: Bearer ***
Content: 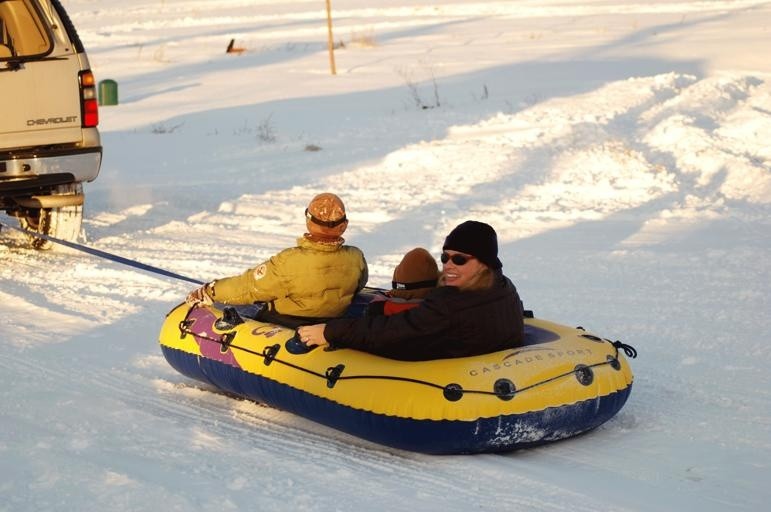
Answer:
[391,248,441,300]
[444,221,498,269]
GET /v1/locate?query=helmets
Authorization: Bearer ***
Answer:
[306,193,348,239]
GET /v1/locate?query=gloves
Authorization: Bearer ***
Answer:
[186,280,216,306]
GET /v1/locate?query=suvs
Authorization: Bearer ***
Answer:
[0,1,102,251]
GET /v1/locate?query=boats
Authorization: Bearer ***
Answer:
[159,276,636,456]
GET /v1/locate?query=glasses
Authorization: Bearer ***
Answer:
[441,253,476,265]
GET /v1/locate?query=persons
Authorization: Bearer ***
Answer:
[297,221,525,361]
[350,247,441,318]
[184,193,368,327]
